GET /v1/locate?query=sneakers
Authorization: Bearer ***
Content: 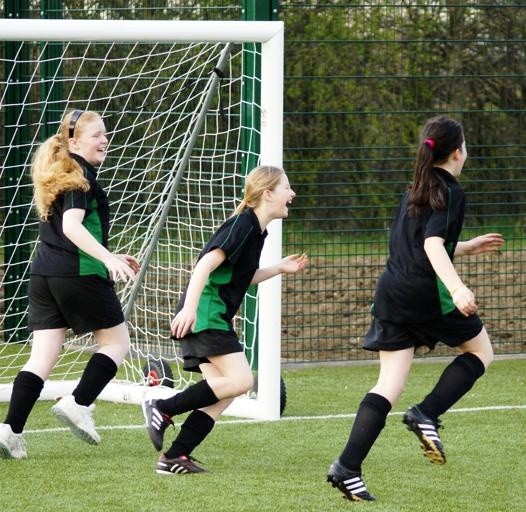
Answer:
[141,397,175,453]
[0,422,28,460]
[155,450,210,477]
[325,457,376,504]
[400,404,447,464]
[51,394,101,445]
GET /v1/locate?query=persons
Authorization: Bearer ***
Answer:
[0,110,140,462]
[325,115,506,503]
[140,163,312,474]
